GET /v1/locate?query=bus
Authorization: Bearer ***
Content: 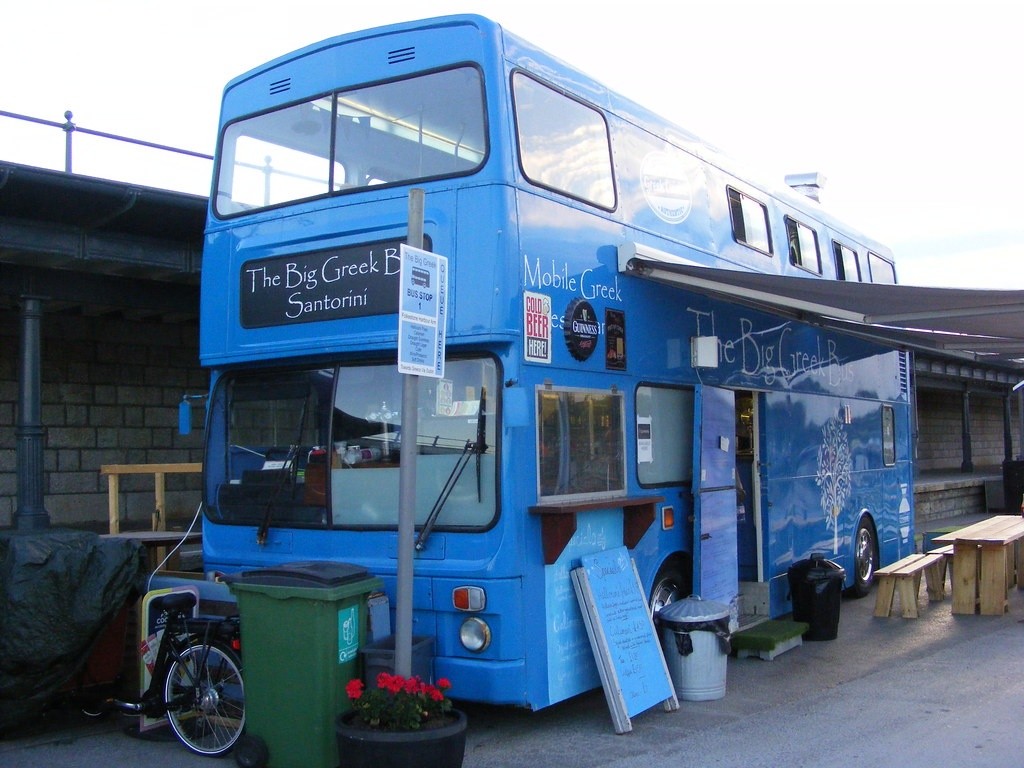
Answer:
[175,11,918,710]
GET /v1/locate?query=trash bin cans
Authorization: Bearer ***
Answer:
[788,554,847,642]
[233,562,384,768]
[659,597,733,702]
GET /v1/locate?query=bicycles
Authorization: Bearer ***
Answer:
[75,590,248,757]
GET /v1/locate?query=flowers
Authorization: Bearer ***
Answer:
[343,671,453,730]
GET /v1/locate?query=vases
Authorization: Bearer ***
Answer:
[335,707,467,768]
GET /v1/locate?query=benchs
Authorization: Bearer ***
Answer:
[927,544,983,593]
[871,553,944,619]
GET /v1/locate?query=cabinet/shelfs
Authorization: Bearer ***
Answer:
[100,463,202,580]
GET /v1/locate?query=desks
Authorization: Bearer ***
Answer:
[931,515,1024,615]
